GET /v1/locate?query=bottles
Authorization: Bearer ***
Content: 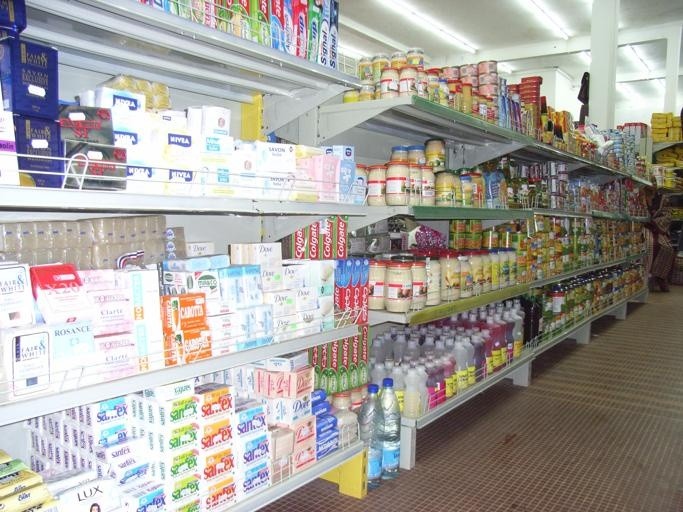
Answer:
[541,96,567,151]
[357,296,543,489]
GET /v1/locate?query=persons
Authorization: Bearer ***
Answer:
[650,190,675,292]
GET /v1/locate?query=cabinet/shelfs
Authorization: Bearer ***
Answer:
[0,0,683,512]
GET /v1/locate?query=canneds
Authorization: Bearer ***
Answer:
[356,48,595,341]
